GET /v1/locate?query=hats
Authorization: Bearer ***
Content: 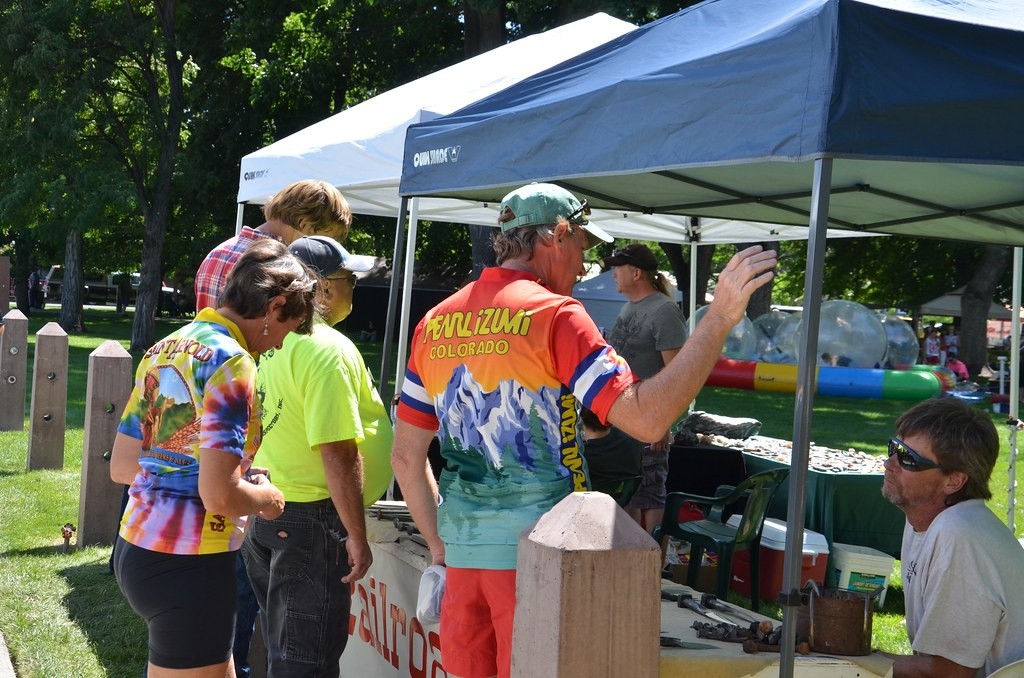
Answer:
[603,244,657,272]
[498,181,614,252]
[287,236,378,277]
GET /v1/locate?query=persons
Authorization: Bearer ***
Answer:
[947,352,970,381]
[922,329,941,365]
[581,407,636,493]
[608,244,688,534]
[923,321,937,339]
[870,397,1024,678]
[389,181,777,678]
[943,324,961,357]
[110,178,397,678]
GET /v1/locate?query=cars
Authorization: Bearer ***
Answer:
[108,271,181,317]
[28,263,119,307]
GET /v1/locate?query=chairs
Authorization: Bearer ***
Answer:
[651,466,790,612]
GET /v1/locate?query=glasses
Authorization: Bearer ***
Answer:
[557,199,592,227]
[888,436,948,473]
[613,247,639,264]
[326,275,356,290]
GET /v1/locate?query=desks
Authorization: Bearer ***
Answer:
[735,434,905,561]
[338,512,894,678]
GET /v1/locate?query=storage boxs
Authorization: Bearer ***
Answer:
[726,514,895,608]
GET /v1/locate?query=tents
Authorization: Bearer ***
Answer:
[387,0,1024,678]
[573,271,683,338]
[236,11,892,413]
[919,285,1012,339]
